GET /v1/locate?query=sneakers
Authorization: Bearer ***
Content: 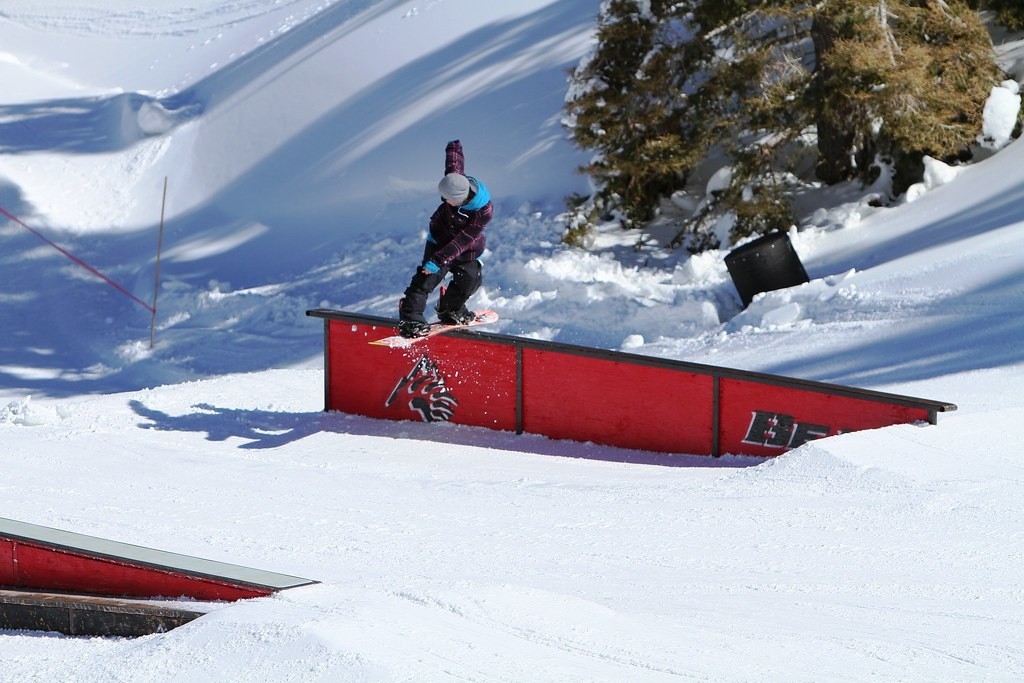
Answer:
[436,286,478,325]
[398,297,431,338]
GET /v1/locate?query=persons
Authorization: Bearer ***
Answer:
[398,138,493,338]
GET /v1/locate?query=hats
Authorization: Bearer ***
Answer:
[438,173,470,203]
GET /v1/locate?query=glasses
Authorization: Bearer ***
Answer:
[440,196,447,202]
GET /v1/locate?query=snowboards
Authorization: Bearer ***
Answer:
[367,309,499,348]
